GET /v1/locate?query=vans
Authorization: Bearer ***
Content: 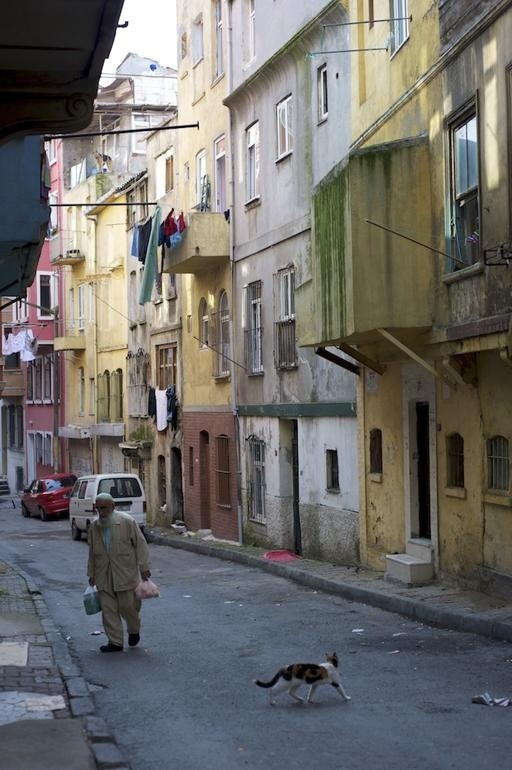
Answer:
[65,474,148,542]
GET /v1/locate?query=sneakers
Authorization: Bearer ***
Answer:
[100,643,123,652]
[128,633,140,646]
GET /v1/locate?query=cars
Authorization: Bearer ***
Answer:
[19,472,82,519]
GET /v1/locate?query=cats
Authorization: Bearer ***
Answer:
[253,652,351,706]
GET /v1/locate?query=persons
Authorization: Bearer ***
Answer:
[86,492,152,654]
